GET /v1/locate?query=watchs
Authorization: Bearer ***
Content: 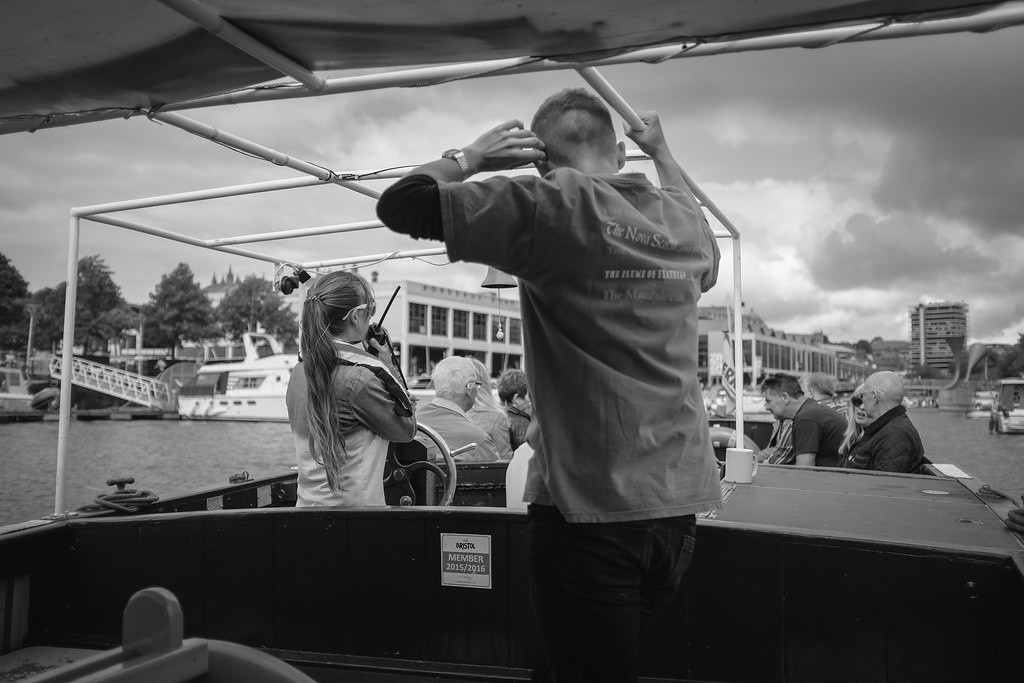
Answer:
[442,148,470,183]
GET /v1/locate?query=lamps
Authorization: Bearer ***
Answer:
[481,266,518,339]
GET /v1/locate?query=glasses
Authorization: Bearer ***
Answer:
[466,380,482,389]
[851,396,864,407]
[341,297,377,321]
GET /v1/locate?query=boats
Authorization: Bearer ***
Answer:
[176,353,303,424]
[965,391,1000,419]
[989,371,1024,435]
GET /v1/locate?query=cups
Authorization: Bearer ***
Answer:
[725,447,758,485]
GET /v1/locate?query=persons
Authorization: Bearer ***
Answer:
[376,87,727,672]
[285,270,416,507]
[756,369,924,473]
[414,354,533,464]
